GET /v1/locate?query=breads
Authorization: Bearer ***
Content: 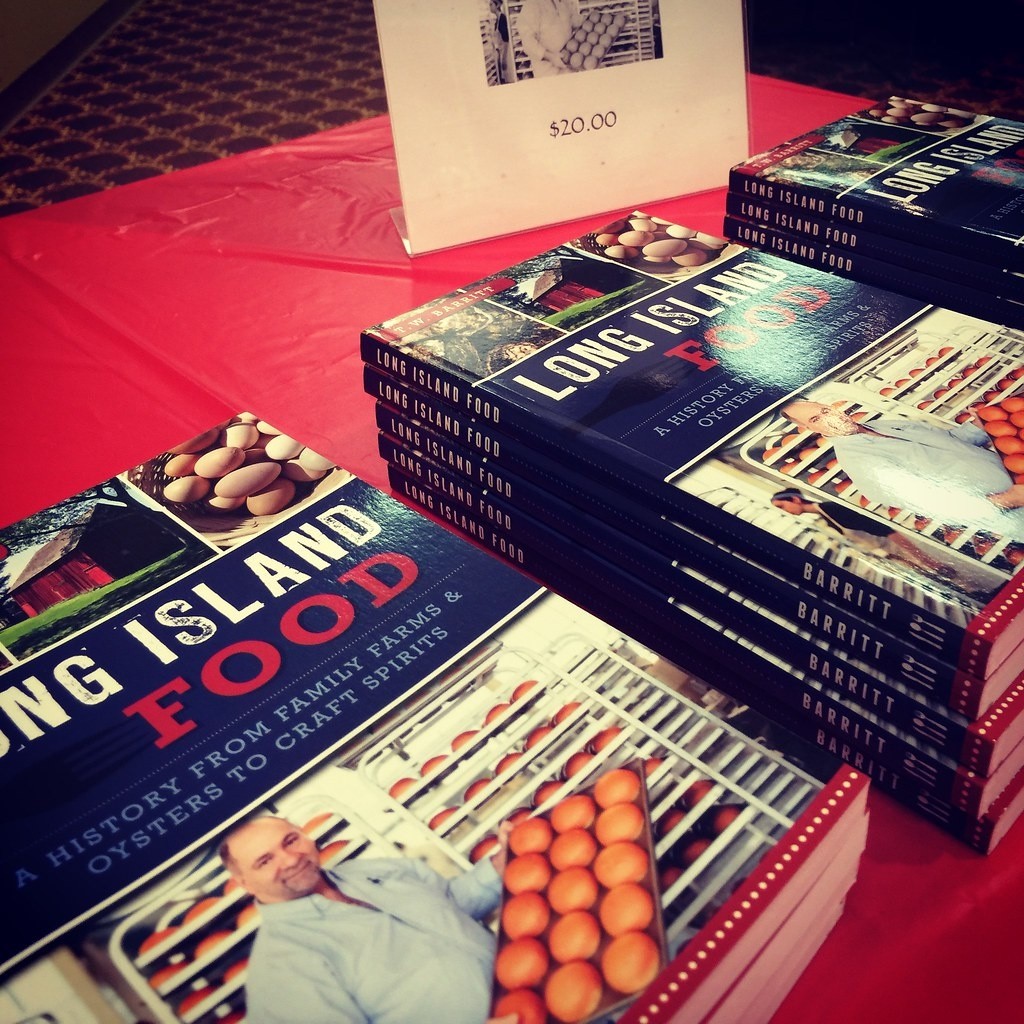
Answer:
[391,675,738,1024]
[137,810,349,1024]
[759,344,1024,569]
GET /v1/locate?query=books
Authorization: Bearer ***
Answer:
[721,95,1024,330]
[359,208,1024,856]
[0,415,870,1024]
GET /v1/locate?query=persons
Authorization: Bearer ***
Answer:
[489,0,604,84]
[781,398,1024,544]
[223,815,517,1024]
[771,487,1005,593]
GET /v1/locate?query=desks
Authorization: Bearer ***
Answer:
[0,68,1023,1024]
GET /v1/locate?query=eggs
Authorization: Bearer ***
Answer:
[869,96,979,128]
[162,411,339,517]
[591,210,728,268]
